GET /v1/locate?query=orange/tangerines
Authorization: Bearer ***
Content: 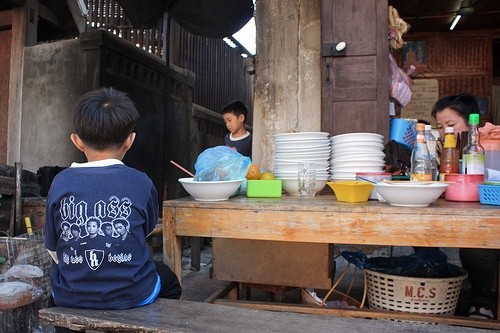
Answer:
[246,164,261,179]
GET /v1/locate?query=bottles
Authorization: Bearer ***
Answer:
[408,113,486,181]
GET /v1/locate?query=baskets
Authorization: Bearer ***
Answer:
[301,251,367,309]
[478,185,500,205]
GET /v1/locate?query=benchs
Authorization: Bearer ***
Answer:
[37,298,500,333]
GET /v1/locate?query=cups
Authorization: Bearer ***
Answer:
[298,162,315,196]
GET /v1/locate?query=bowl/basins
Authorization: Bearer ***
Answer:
[445,174,484,201]
[178,177,244,202]
[326,181,373,203]
[273,131,387,198]
[374,180,448,207]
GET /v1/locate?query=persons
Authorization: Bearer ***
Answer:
[45,85,181,333]
[223,100,253,160]
[413,91,500,324]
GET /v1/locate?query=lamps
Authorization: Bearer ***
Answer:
[449,14,461,30]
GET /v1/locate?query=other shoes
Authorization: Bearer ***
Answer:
[466,305,494,321]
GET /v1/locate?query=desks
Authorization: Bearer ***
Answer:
[162,194,500,301]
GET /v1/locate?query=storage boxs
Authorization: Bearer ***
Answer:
[21,197,53,231]
[246,179,282,198]
[479,139,500,181]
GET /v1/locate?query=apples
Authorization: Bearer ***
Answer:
[259,171,275,180]
